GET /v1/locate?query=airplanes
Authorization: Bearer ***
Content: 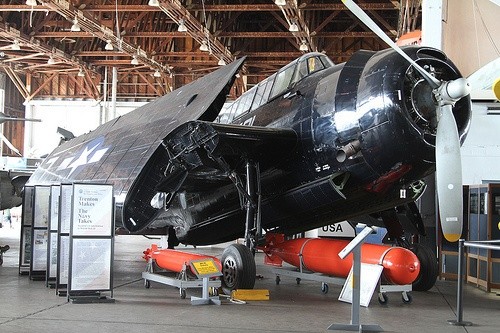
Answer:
[12,0,498,292]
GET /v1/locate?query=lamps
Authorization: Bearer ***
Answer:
[26,0,308,77]
